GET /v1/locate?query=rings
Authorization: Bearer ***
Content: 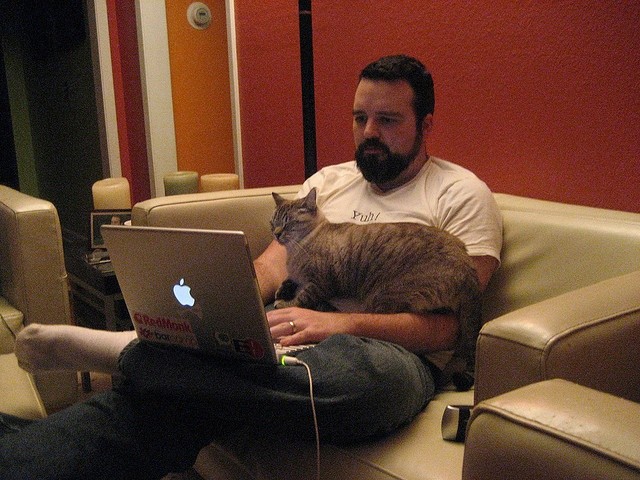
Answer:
[289,321,297,335]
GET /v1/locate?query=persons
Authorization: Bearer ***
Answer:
[0,55,502,480]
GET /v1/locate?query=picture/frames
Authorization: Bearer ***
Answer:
[90,208,133,252]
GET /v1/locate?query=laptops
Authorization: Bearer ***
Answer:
[99,223,318,367]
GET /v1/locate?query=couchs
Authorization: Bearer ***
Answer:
[1,184,79,420]
[134,183,639,480]
[462,374,639,480]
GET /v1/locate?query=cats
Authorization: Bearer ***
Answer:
[268,186,486,393]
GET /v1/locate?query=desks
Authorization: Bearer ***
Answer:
[63,259,134,393]
